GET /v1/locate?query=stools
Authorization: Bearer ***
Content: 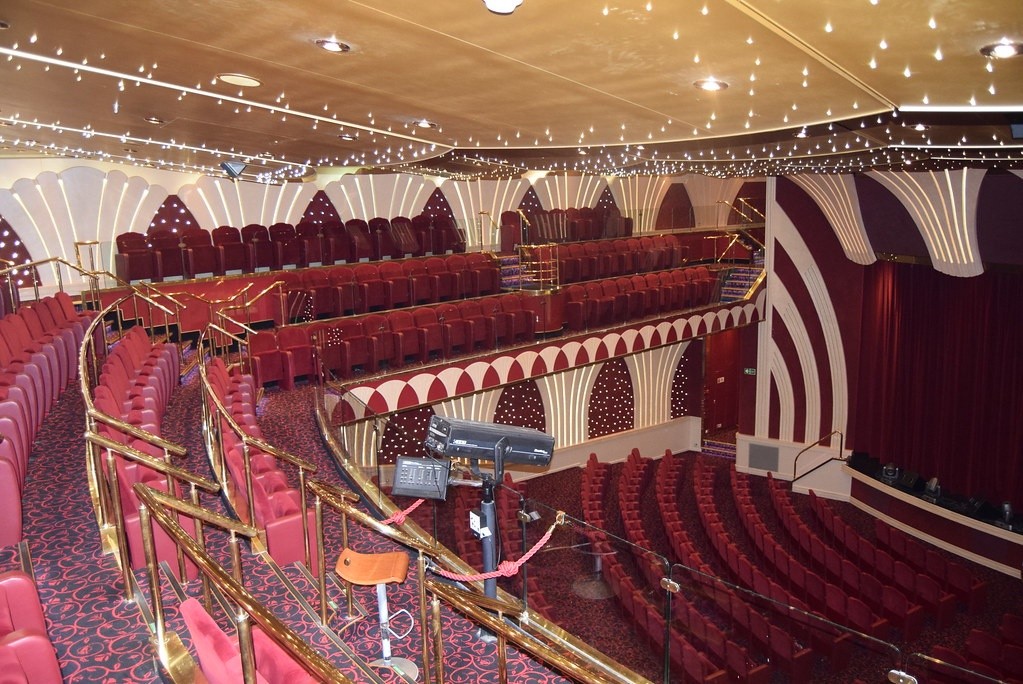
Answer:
[336,548,418,681]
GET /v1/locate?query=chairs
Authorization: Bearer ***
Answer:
[0,207,1023,684]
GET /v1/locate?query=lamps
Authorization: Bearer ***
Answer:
[995,502,1013,530]
[882,463,899,485]
[922,477,940,503]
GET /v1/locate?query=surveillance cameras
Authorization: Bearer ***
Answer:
[424,415,555,466]
